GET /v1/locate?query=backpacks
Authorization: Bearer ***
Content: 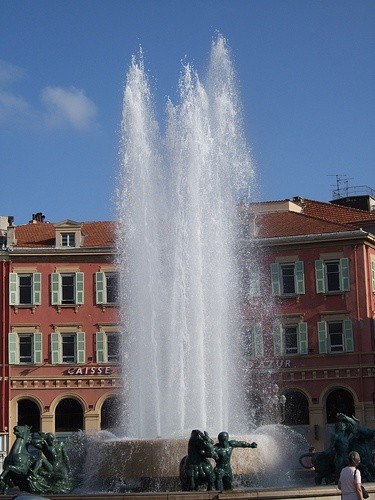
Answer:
[353,470,370,499]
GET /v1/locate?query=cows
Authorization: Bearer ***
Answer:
[299,415,375,484]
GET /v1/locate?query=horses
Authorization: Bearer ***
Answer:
[0,424,81,495]
[179,430,215,491]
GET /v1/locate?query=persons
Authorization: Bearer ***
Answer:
[28,432,73,490]
[338,452,366,500]
[211,432,258,491]
[329,413,375,483]
